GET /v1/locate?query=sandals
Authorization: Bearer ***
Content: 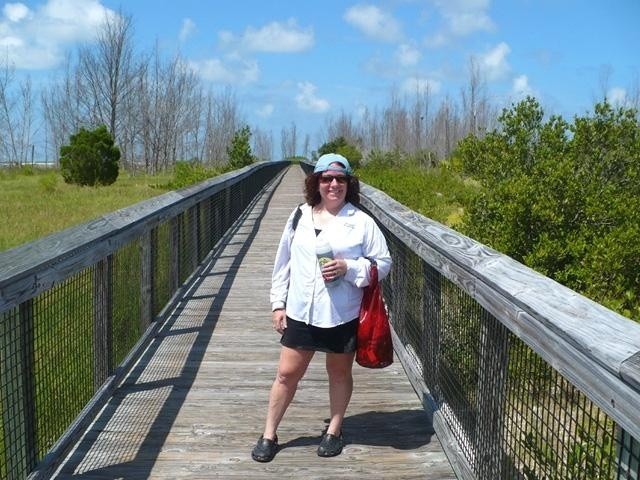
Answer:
[252,433,278,462]
[317,427,343,457]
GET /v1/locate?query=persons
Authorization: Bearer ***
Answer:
[251,152,392,465]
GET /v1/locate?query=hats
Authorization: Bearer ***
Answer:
[313,153,351,175]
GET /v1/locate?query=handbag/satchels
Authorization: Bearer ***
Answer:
[355,258,393,368]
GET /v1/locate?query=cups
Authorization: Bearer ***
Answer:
[315,248,341,289]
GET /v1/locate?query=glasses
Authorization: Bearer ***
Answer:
[317,174,349,184]
